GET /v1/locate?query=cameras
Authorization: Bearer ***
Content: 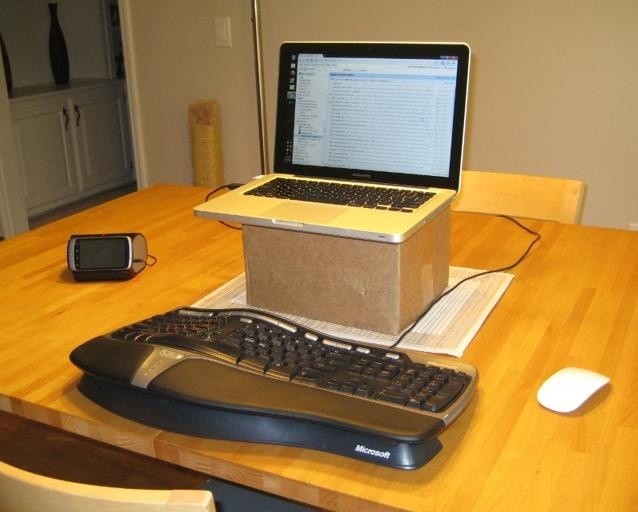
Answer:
[65,232,149,281]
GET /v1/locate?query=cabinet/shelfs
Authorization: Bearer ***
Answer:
[10,79,136,219]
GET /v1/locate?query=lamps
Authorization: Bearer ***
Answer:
[48,3,69,84]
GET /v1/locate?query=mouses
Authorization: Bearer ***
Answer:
[534,364,612,415]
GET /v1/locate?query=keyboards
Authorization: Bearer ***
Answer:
[67,303,480,445]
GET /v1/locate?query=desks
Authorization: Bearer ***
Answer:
[0,180,638,512]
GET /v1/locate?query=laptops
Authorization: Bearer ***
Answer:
[191,39,472,245]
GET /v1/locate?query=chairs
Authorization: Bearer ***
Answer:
[450,170,586,225]
[0,460,216,512]
